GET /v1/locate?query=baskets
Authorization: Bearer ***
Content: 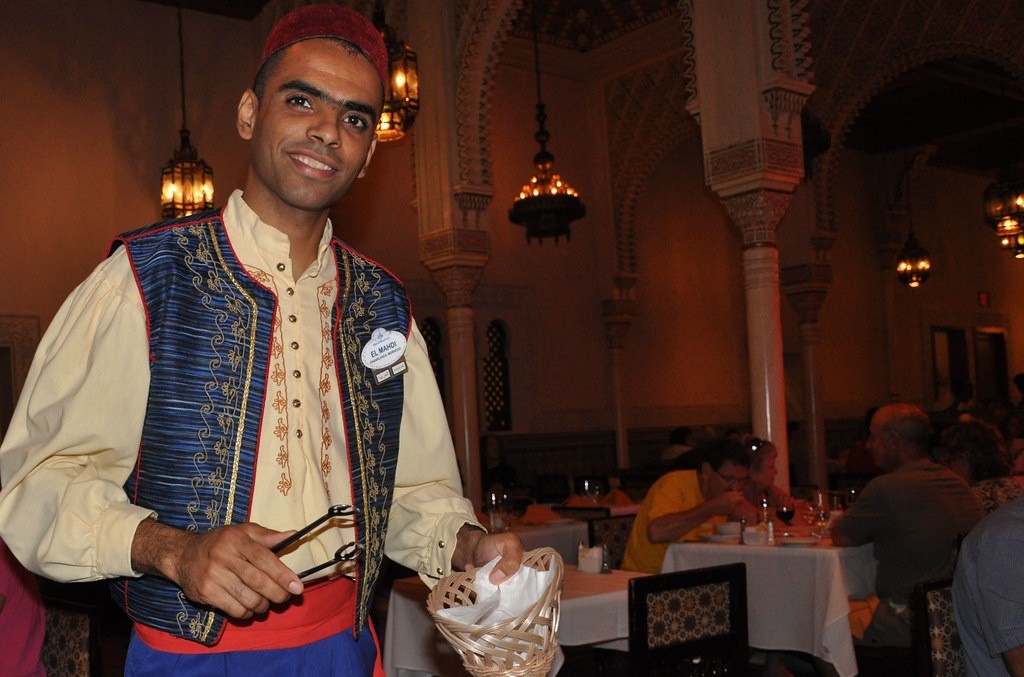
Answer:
[426,545,565,677]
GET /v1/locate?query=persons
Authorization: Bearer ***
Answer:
[480,373,1024,677]
[0,538,48,677]
[0,2,524,677]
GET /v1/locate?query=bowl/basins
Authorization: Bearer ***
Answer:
[715,521,741,533]
[742,532,766,544]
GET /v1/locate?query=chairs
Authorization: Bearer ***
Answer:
[482,472,646,571]
[846,530,971,677]
[39,595,104,677]
[556,561,749,677]
[789,481,820,504]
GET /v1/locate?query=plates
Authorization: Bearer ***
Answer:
[700,533,740,543]
[783,541,819,547]
[544,519,575,526]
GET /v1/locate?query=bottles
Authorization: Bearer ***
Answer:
[765,520,776,545]
[489,493,511,533]
[602,544,610,573]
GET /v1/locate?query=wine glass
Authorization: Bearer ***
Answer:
[757,497,795,537]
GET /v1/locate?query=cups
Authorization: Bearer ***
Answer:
[800,492,830,538]
[589,483,603,506]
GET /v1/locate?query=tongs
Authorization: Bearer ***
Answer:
[268,503,365,578]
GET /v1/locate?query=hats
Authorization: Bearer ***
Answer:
[258,2,388,102]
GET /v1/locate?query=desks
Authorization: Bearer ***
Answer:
[385,503,879,677]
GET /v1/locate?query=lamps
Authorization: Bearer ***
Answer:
[370,0,420,141]
[896,148,932,290]
[982,66,1024,259]
[159,0,215,221]
[507,0,588,248]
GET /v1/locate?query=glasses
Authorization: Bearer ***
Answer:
[711,463,752,485]
[751,437,764,456]
